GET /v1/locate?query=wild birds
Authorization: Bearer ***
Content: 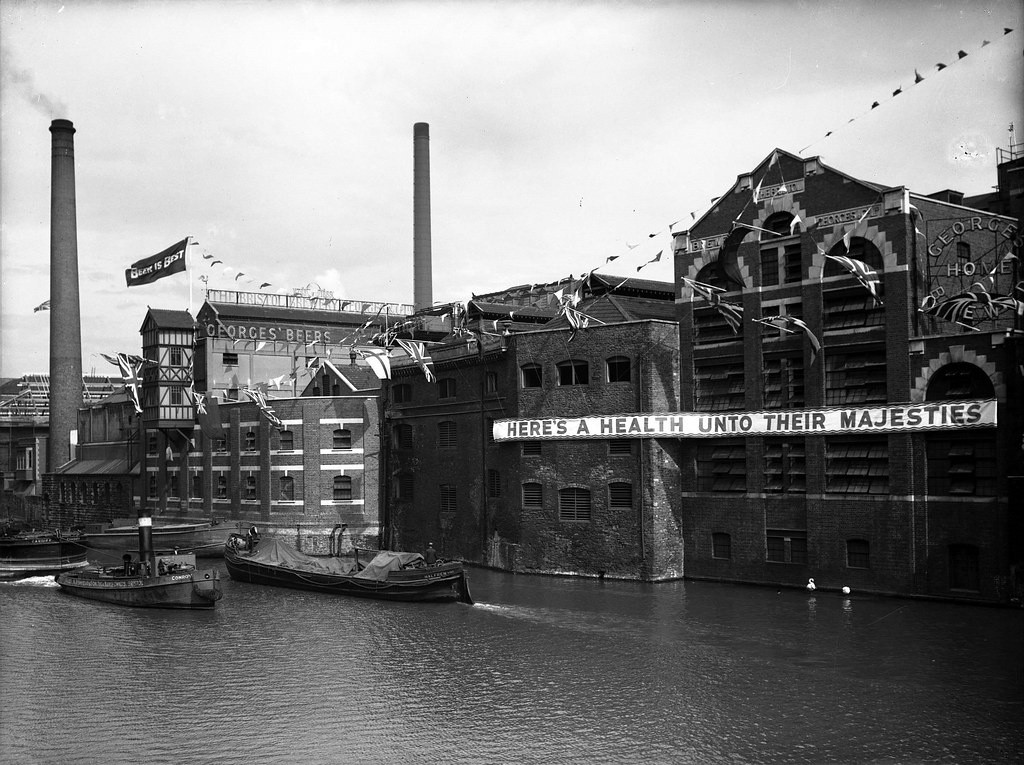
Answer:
[842,586,851,594]
[806,577,816,590]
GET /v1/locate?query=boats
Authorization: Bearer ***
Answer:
[0,495,232,610]
[226,522,477,606]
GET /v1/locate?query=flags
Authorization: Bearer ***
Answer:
[124,237,188,286]
[758,315,821,367]
[680,277,744,339]
[33,299,50,313]
[244,391,282,428]
[396,340,437,384]
[357,348,392,380]
[99,352,150,418]
[720,226,755,288]
[193,394,209,415]
[563,308,590,342]
[917,292,1023,332]
[822,252,884,308]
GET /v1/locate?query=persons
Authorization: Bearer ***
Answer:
[425,542,437,566]
[122,550,131,575]
